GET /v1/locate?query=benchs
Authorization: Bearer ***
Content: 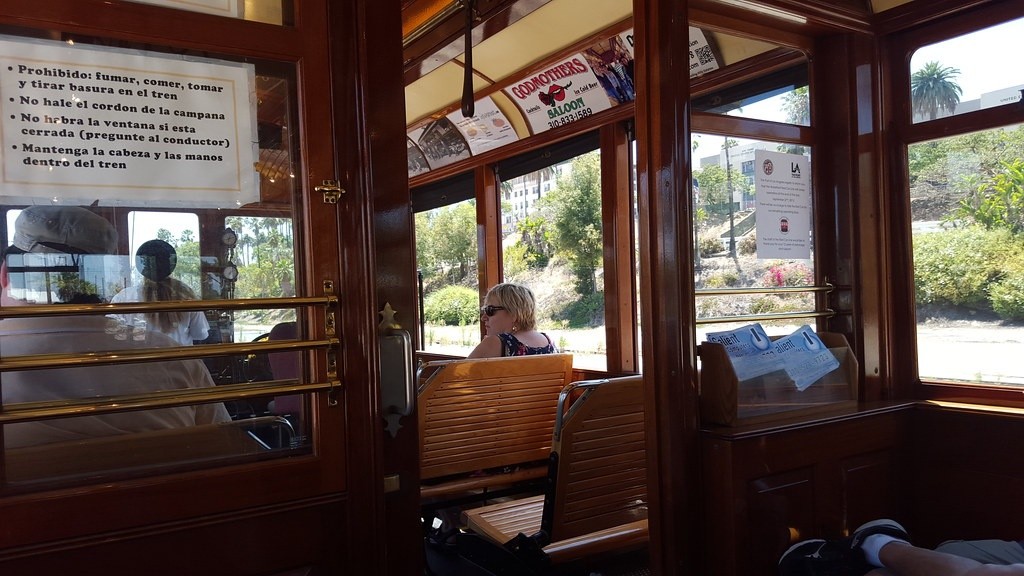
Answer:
[416,352,574,550]
[4,415,296,481]
[461,374,650,576]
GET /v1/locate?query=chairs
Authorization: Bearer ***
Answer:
[266,322,301,448]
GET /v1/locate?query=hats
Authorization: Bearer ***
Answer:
[135,240,177,280]
[14,205,119,253]
[597,62,605,67]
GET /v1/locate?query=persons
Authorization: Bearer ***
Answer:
[431,284,552,545]
[107,240,210,346]
[590,49,635,104]
[778,519,1024,576]
[265,323,300,413]
[414,159,420,172]
[1,205,231,446]
[425,135,451,159]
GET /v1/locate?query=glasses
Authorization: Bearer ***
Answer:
[480,305,510,316]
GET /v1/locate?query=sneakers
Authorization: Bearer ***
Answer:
[778,518,912,576]
